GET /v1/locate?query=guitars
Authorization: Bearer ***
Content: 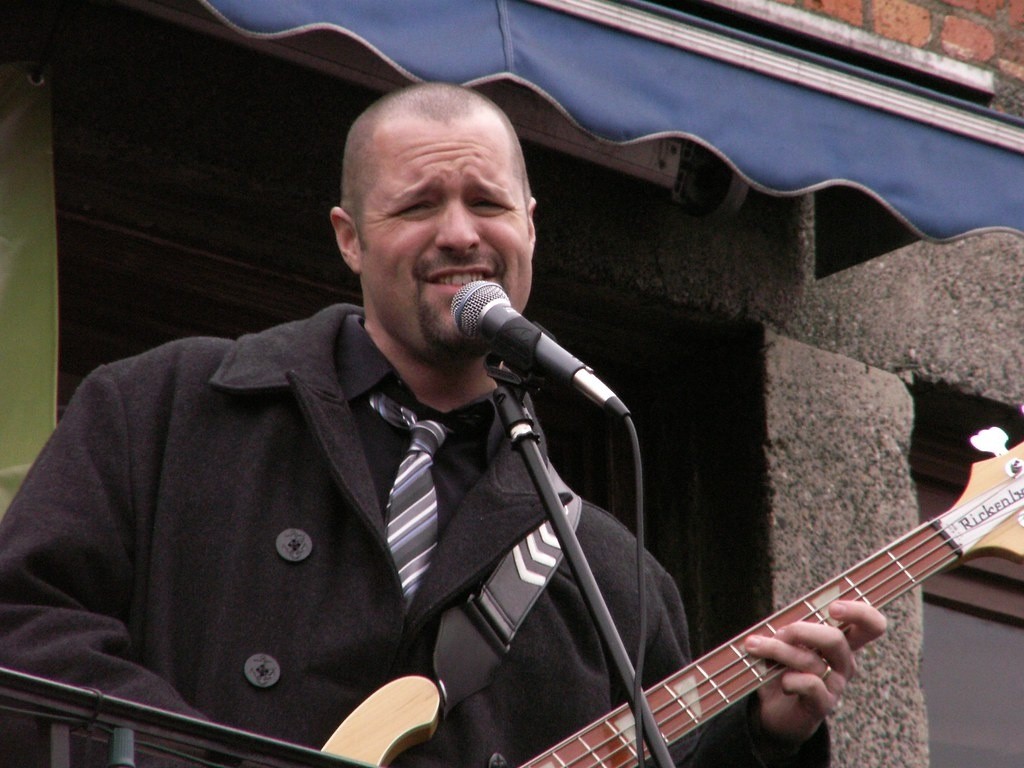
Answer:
[314,419,1024,767]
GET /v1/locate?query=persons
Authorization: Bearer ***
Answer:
[0,82,888,768]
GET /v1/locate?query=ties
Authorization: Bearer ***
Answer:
[368,390,454,616]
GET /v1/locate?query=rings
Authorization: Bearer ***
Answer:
[821,665,832,681]
